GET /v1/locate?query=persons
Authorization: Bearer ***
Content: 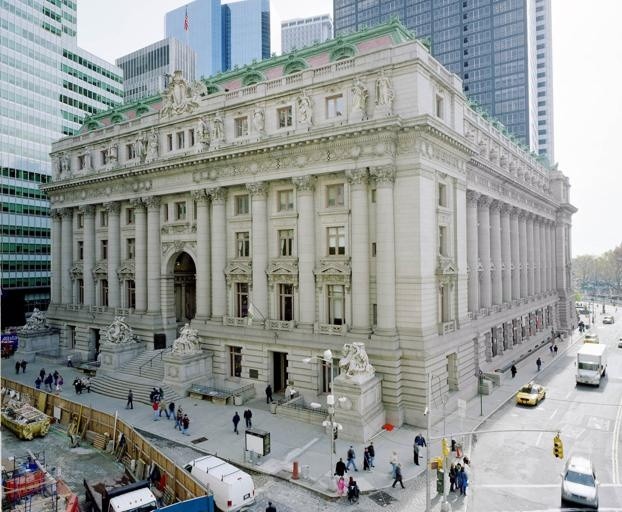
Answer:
[415,433,427,458]
[35,367,64,393]
[232,411,240,436]
[337,341,374,376]
[150,387,190,434]
[244,408,253,428]
[296,90,312,123]
[4,341,10,359]
[391,462,405,490]
[536,357,542,373]
[265,500,276,512]
[265,385,273,404]
[252,102,266,131]
[170,322,201,356]
[57,111,223,177]
[350,76,369,110]
[21,359,27,374]
[373,70,395,107]
[414,439,420,465]
[334,441,375,505]
[578,302,605,334]
[448,455,471,497]
[390,451,399,479]
[548,332,565,357]
[23,307,50,330]
[289,387,296,401]
[73,376,92,395]
[15,361,20,374]
[104,316,136,345]
[510,363,517,380]
[67,354,73,367]
[124,389,134,409]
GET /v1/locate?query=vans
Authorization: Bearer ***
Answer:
[1,340,14,357]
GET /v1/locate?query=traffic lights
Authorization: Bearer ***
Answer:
[552,439,564,459]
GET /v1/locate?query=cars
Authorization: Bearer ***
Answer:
[618,337,622,348]
[516,381,546,406]
[603,316,614,325]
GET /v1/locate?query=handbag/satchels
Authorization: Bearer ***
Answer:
[449,473,455,478]
[458,480,462,488]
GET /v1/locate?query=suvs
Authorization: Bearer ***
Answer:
[585,334,600,344]
[559,456,599,509]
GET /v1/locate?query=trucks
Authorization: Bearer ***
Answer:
[572,343,610,387]
[84,467,158,512]
[183,455,257,512]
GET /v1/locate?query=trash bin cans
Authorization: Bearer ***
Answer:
[302,465,311,481]
[271,401,278,413]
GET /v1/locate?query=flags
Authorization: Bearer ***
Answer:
[184,12,190,33]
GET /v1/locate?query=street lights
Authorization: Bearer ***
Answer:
[303,350,349,491]
